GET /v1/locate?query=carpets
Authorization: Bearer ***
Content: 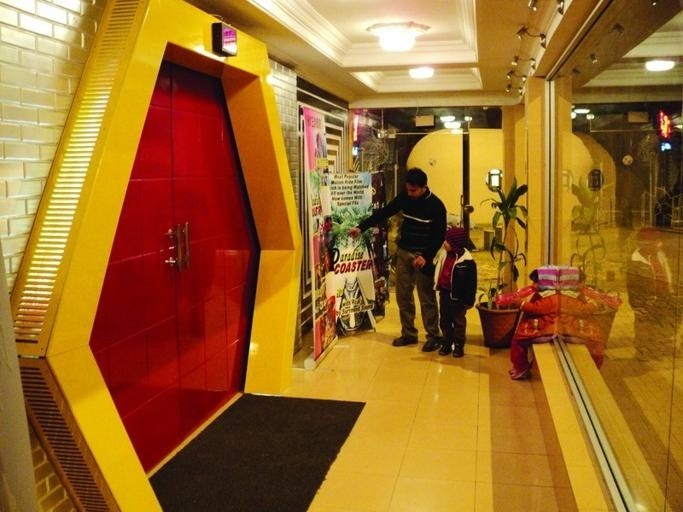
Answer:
[147,390,365,510]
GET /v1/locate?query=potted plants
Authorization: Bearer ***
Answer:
[475,173,530,348]
[564,174,618,369]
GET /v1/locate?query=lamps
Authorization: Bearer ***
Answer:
[503,0,566,98]
[372,14,465,135]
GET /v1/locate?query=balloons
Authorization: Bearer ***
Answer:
[580,287,621,309]
[495,285,536,305]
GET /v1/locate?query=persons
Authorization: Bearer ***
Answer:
[627,226,676,360]
[347,168,447,352]
[508,264,559,381]
[417,227,477,358]
[559,266,604,370]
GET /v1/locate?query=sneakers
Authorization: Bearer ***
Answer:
[508,363,530,380]
[392,336,418,346]
[422,340,464,357]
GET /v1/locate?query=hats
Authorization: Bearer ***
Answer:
[444,228,466,255]
[637,228,661,255]
